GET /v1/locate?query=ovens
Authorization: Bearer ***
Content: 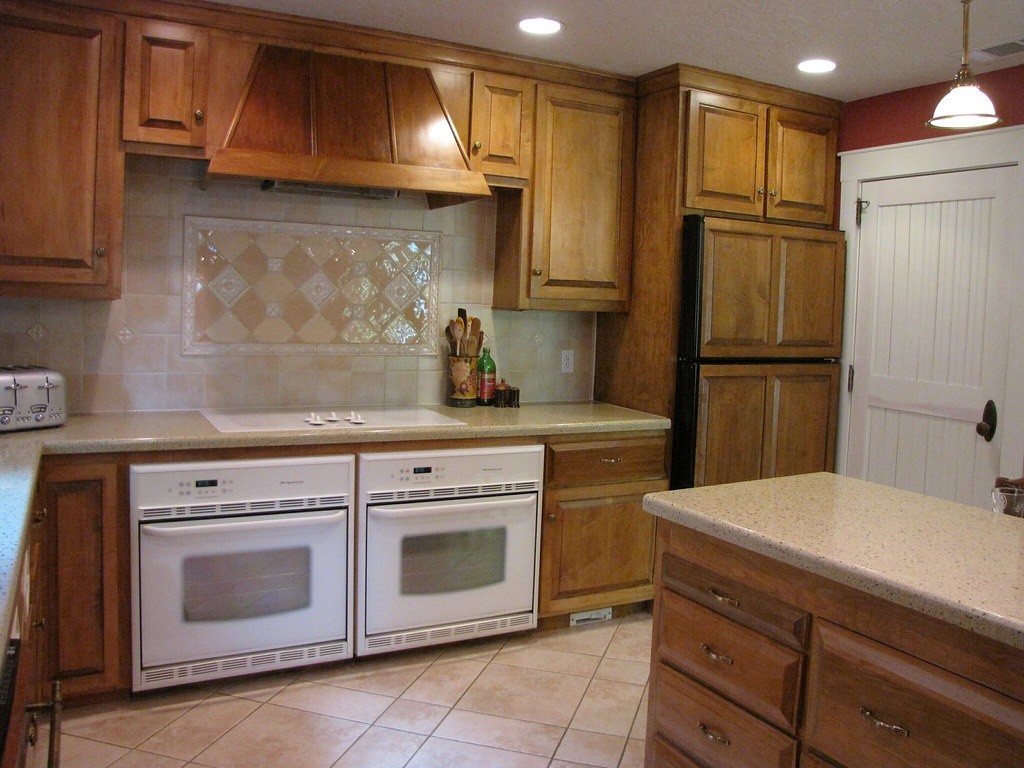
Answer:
[129,441,545,694]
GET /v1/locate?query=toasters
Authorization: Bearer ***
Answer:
[0,364,67,431]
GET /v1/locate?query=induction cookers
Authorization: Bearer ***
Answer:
[198,404,468,433]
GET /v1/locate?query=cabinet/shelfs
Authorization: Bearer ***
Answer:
[537,429,671,632]
[592,63,846,492]
[118,16,211,160]
[0,453,126,768]
[491,79,637,313]
[0,0,124,300]
[643,515,1024,768]
[468,70,533,192]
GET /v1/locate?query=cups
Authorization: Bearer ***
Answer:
[507,386,520,407]
[446,355,478,407]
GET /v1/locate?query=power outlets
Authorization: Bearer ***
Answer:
[561,350,574,374]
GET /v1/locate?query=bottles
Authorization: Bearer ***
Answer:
[494,378,509,408]
[476,347,497,405]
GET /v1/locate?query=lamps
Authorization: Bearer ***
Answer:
[923,0,1003,131]
